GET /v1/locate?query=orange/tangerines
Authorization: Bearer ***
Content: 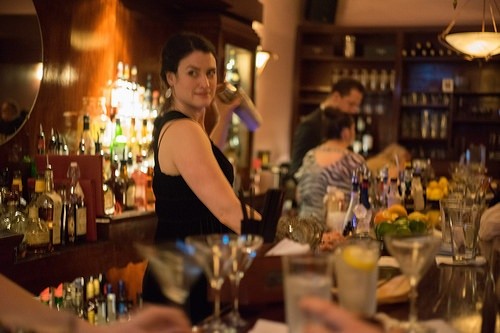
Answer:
[426,177,450,200]
[374,204,443,238]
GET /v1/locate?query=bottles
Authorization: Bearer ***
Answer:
[331,67,395,91]
[29,272,129,325]
[363,93,385,115]
[1,161,88,257]
[35,123,68,157]
[74,61,158,216]
[352,117,375,153]
[324,159,432,236]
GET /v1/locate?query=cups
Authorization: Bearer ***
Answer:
[229,84,263,130]
[438,160,489,264]
[335,237,381,320]
[282,253,332,332]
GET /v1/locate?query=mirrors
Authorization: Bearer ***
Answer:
[0,0,43,145]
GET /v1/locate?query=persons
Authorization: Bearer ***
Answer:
[298,297,454,333]
[142,32,266,327]
[477,146,500,299]
[280,77,367,184]
[0,276,192,333]
[294,106,372,223]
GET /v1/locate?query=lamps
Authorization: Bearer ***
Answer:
[438,0,500,62]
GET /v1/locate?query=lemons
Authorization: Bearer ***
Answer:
[343,244,378,270]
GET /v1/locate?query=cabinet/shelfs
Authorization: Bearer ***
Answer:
[290,23,500,176]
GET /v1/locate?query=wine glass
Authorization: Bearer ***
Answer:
[135,234,264,332]
[382,226,441,332]
